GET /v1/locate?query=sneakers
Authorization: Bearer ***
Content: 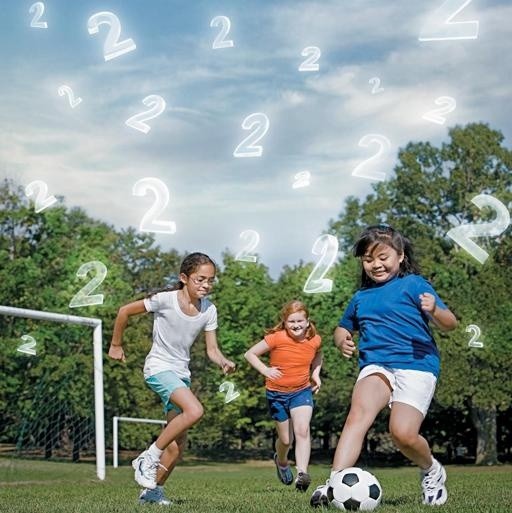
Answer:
[132,450,157,490]
[419,461,447,505]
[295,472,311,491]
[274,453,293,484]
[140,488,172,506]
[310,484,328,505]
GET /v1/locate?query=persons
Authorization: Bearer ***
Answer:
[310,224,459,510]
[244,301,324,490]
[108,253,238,506]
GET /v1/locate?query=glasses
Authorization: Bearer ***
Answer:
[189,277,216,285]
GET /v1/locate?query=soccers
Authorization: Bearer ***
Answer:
[327,466,383,513]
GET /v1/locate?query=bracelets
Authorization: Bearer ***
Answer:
[427,306,436,314]
